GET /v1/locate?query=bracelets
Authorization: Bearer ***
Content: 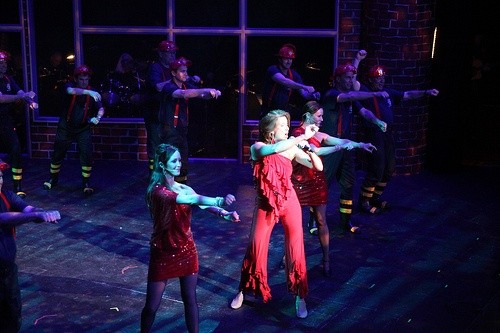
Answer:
[377,120,381,126]
[301,134,308,141]
[214,197,224,207]
[360,142,365,151]
[216,208,228,220]
[96,115,102,119]
[335,144,342,151]
[425,89,426,94]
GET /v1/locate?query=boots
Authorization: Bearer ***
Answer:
[357,184,378,214]
[43,163,62,190]
[11,168,26,199]
[340,195,360,233]
[307,207,319,236]
[81,164,93,194]
[175,176,187,184]
[148,159,154,178]
[369,180,389,210]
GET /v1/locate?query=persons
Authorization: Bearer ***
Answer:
[0,51,39,199]
[231,110,324,318]
[39,48,68,115]
[257,44,323,120]
[0,157,61,333]
[120,53,136,76]
[43,65,105,193]
[156,57,221,185]
[351,50,438,214]
[279,101,378,272]
[139,41,202,179]
[140,144,240,333]
[308,61,390,235]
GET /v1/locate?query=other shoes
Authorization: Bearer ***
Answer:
[279,256,286,269]
[295,296,308,318]
[323,262,330,274]
[231,290,245,309]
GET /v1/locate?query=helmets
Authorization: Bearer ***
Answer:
[279,46,297,58]
[171,57,191,69]
[368,66,386,78]
[160,41,180,51]
[0,159,10,171]
[334,62,356,74]
[0,52,8,61]
[284,44,296,50]
[74,65,93,75]
